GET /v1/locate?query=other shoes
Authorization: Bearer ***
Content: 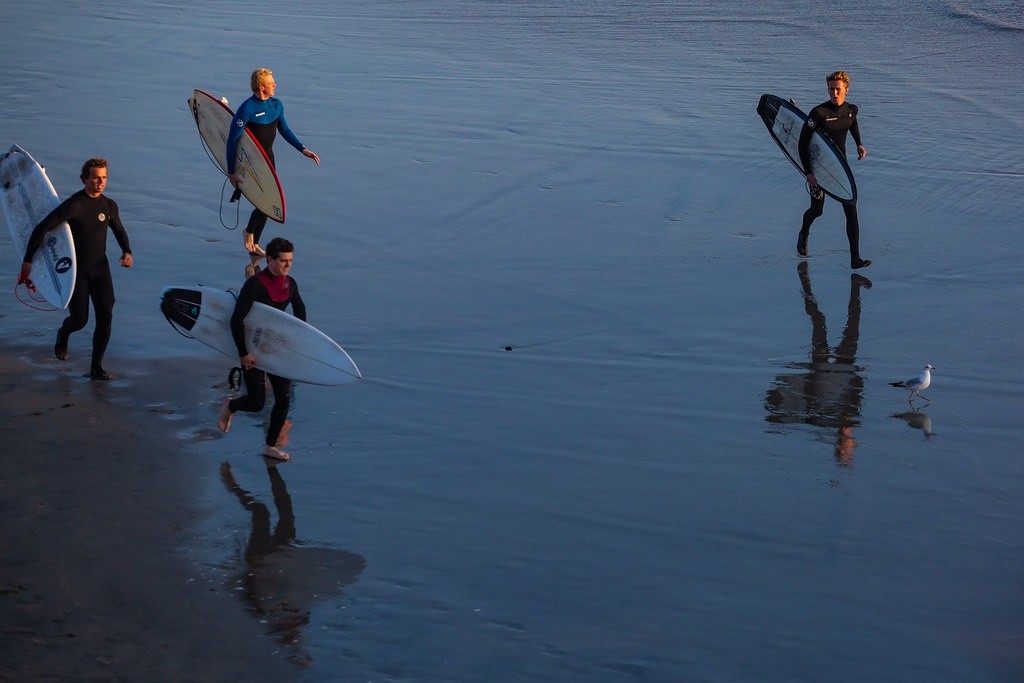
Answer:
[797,231,808,255]
[797,254,810,272]
[851,260,871,268]
[89,368,110,381]
[54,327,69,360]
[851,273,873,289]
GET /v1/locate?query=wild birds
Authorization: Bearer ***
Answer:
[887,364,936,401]
[890,401,938,439]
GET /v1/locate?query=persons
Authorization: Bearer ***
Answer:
[220,453,313,669]
[218,237,307,460]
[796,71,871,269]
[18,159,133,380]
[245,252,265,279]
[226,67,321,255]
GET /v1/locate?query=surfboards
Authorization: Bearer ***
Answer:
[186,88,286,225]
[157,286,363,387]
[0,143,78,312]
[756,93,858,206]
